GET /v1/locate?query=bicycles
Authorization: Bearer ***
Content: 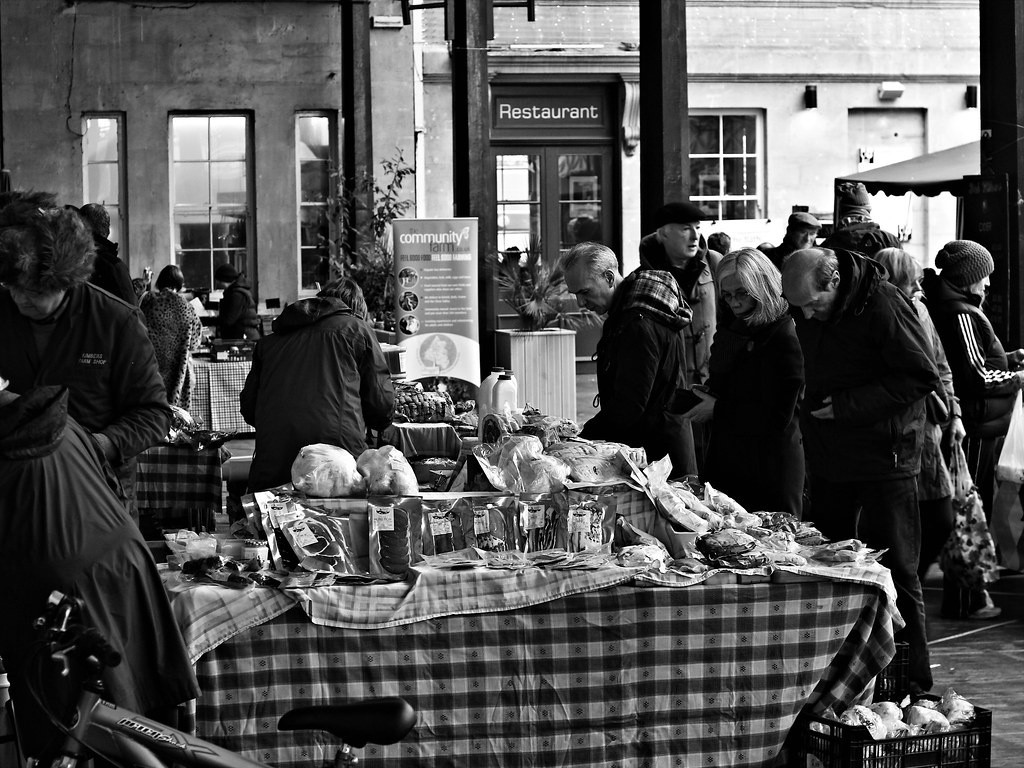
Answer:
[0,589,417,768]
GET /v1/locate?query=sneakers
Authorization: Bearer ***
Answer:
[968,607,1001,620]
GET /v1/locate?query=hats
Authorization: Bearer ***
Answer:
[660,202,707,224]
[215,263,236,282]
[935,240,994,288]
[839,183,872,214]
[788,212,822,229]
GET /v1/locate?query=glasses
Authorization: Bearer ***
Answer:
[722,292,748,300]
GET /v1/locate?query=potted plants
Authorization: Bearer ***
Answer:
[309,142,416,373]
[485,238,609,425]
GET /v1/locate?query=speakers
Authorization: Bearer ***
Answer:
[967,85,977,108]
[806,84,816,108]
[879,81,904,99]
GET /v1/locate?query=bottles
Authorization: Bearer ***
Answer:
[478,366,517,445]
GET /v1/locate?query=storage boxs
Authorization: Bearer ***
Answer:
[209,339,257,362]
[787,694,993,768]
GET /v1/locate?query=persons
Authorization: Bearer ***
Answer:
[757,212,822,271]
[679,247,806,519]
[920,240,1024,619]
[782,246,940,693]
[874,247,967,577]
[0,374,201,729]
[214,264,259,341]
[0,196,174,492]
[707,233,730,256]
[639,202,725,388]
[562,242,698,481]
[239,276,395,494]
[820,190,903,260]
[76,203,136,304]
[137,265,203,410]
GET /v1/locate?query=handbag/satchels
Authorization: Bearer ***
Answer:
[938,442,1001,585]
[995,387,1024,484]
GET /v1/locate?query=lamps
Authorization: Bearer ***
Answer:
[804,86,817,108]
[965,86,976,107]
[878,81,905,100]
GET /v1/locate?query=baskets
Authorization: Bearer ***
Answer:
[875,639,909,702]
[796,693,992,768]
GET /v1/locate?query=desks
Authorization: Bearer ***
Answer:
[136,358,905,767]
